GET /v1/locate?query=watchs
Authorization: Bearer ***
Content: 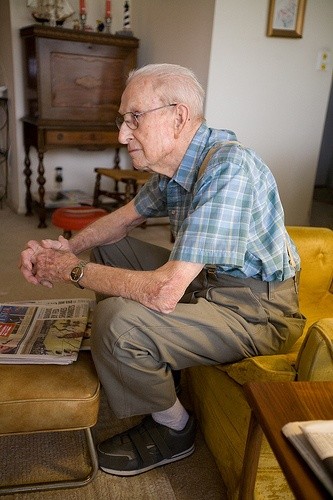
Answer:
[69,259,93,290]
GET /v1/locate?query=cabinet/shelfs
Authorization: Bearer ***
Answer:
[19,24,140,123]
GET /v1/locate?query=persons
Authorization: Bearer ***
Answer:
[21,62,305,477]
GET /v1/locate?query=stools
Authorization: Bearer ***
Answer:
[51,206,108,240]
[0,349,101,497]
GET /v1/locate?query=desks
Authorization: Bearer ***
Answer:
[91,167,162,229]
[19,117,136,230]
[236,381,333,500]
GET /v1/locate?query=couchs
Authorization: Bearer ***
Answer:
[170,224,333,500]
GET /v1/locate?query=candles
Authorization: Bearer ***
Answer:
[78,0,86,16]
[105,0,111,16]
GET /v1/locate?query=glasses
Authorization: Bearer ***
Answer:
[116,104,181,130]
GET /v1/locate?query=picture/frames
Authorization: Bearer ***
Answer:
[266,0,307,38]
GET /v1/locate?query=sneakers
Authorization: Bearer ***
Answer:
[96,414,195,476]
